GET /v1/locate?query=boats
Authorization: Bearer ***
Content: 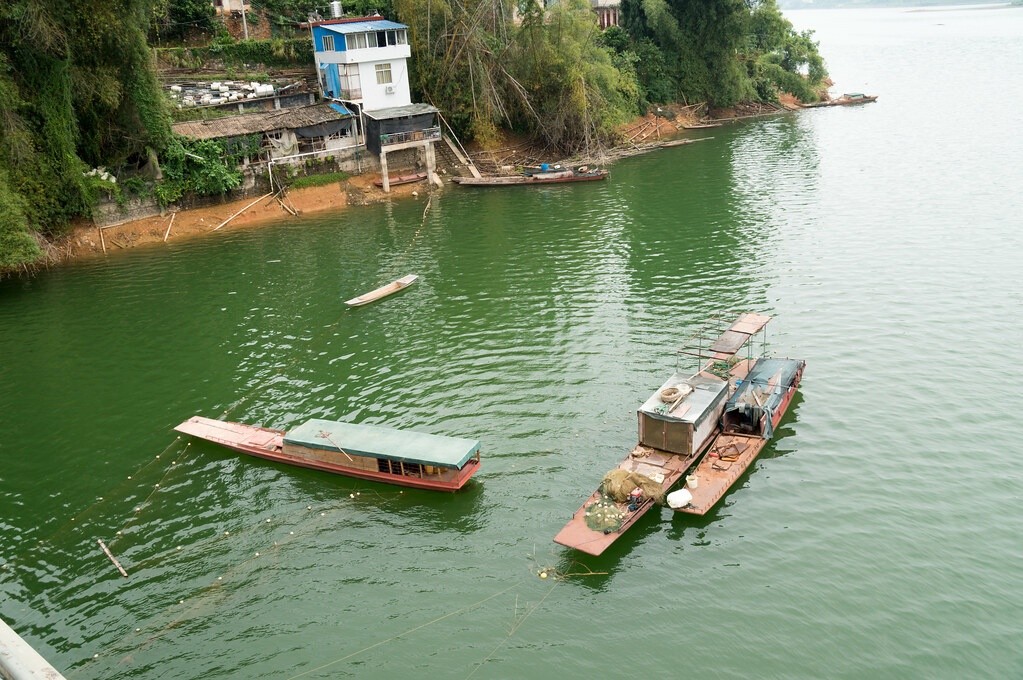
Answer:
[450,162,607,188]
[671,355,808,518]
[374,171,428,186]
[343,273,420,307]
[172,415,482,492]
[794,92,879,109]
[551,313,774,557]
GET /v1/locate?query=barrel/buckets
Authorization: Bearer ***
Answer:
[686,474,698,489]
[666,488,692,509]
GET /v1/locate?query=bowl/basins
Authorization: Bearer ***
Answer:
[660,388,680,402]
